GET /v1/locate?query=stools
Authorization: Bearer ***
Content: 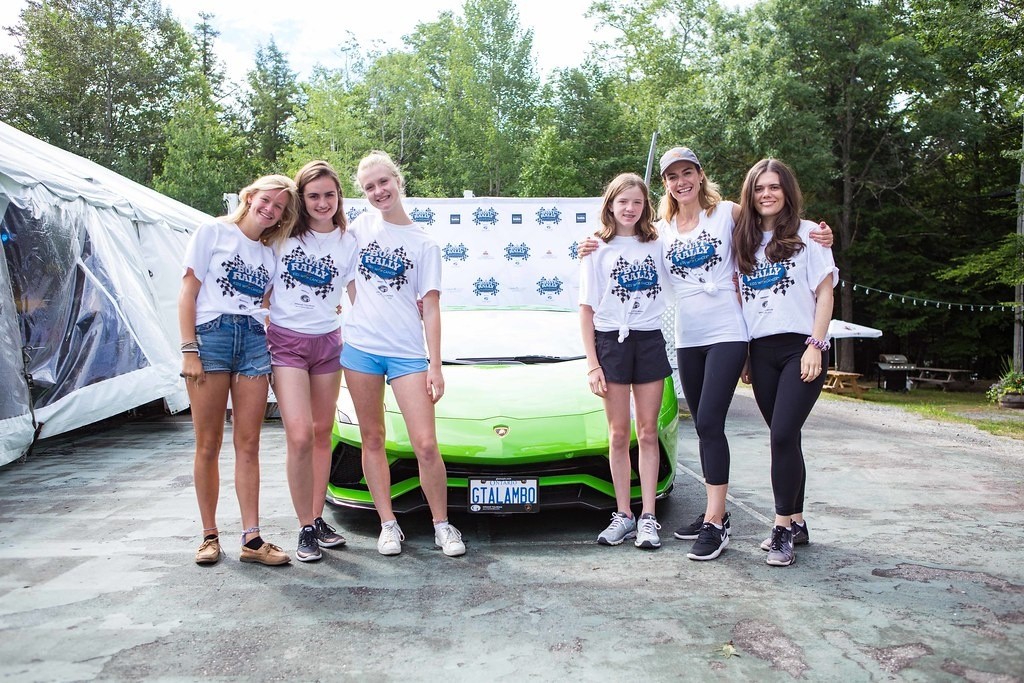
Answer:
[822,381,873,394]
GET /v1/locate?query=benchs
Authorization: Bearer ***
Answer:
[907,376,955,391]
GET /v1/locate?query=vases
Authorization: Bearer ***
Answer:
[997,389,1024,407]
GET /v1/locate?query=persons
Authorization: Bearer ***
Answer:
[178,173,343,565]
[730,159,840,566]
[577,147,833,560]
[579,172,740,548]
[336,150,466,556]
[260,160,424,561]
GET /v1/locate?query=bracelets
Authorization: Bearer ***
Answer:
[587,366,601,376]
[180,342,200,353]
[806,337,828,351]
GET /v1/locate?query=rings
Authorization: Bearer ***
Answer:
[189,377,192,380]
[819,368,822,370]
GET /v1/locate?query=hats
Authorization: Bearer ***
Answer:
[659,147,702,176]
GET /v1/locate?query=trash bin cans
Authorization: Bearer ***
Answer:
[878,355,908,391]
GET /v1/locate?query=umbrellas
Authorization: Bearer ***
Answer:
[825,318,882,371]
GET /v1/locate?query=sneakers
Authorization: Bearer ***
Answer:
[296,525,322,561]
[378,521,404,555]
[597,512,638,546]
[687,515,729,560]
[240,543,291,565]
[313,517,346,547]
[196,537,220,562]
[674,512,731,539]
[634,513,662,547]
[766,525,795,566]
[760,519,809,550]
[434,525,466,555]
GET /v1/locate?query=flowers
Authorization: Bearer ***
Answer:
[1000,373,1024,391]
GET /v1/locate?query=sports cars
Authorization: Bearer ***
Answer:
[326,307,680,514]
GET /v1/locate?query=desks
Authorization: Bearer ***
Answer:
[913,367,971,391]
[827,370,864,398]
